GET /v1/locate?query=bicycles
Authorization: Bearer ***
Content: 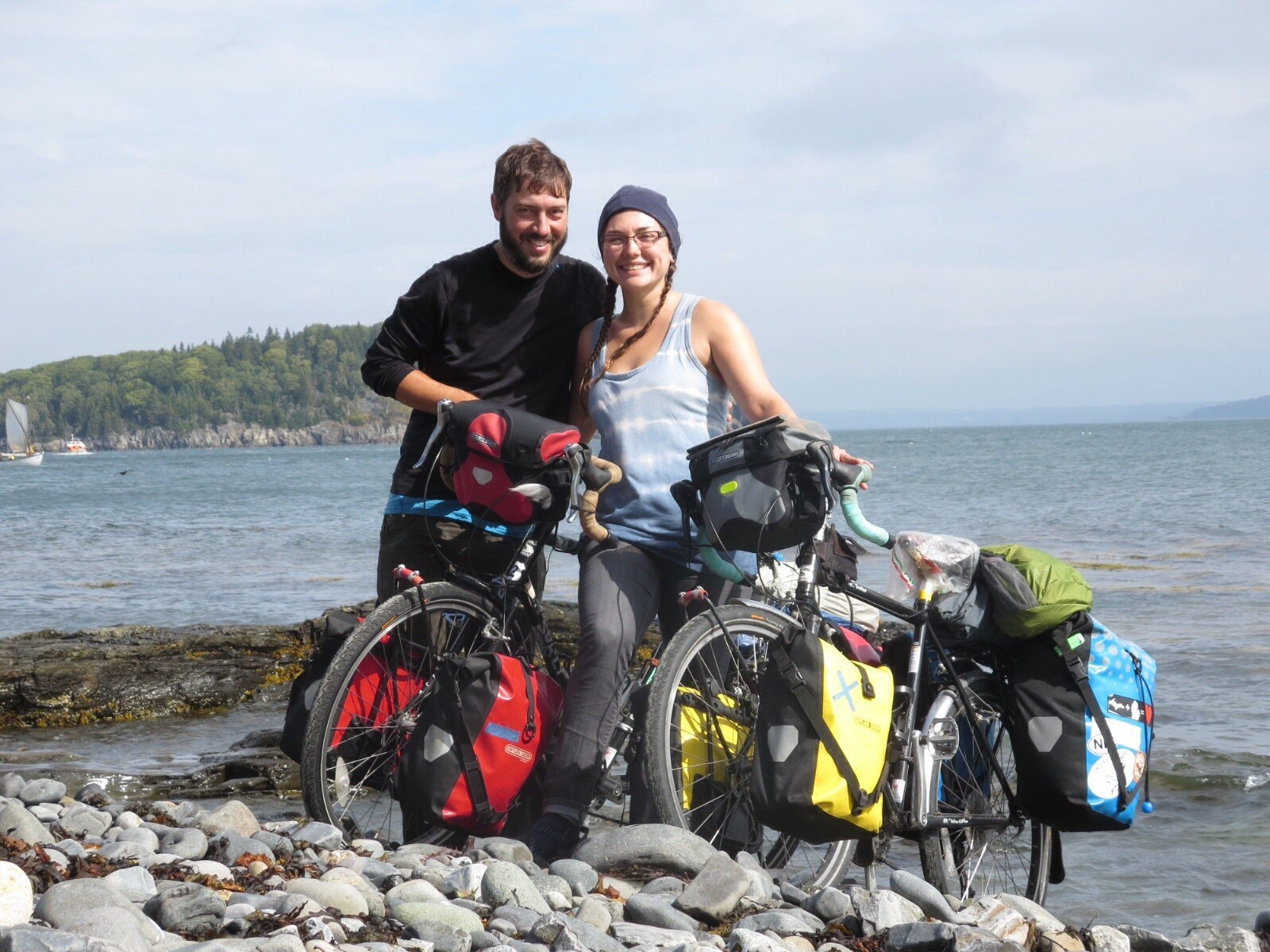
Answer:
[299,399,802,876]
[634,445,1072,912]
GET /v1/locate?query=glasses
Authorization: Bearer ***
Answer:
[602,230,667,248]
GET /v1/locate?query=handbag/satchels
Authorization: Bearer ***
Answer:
[277,609,564,839]
[980,543,1093,635]
[749,623,894,845]
[627,680,759,855]
[1014,610,1157,835]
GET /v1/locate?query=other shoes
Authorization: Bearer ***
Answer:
[518,813,586,864]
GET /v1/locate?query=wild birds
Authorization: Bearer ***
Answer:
[113,469,134,477]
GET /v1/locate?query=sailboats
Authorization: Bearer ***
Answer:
[0,397,46,467]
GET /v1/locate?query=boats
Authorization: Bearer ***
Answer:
[60,434,90,456]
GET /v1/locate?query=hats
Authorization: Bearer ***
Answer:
[597,184,683,260]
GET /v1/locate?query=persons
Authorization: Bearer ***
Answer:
[358,139,613,849]
[508,187,876,869]
